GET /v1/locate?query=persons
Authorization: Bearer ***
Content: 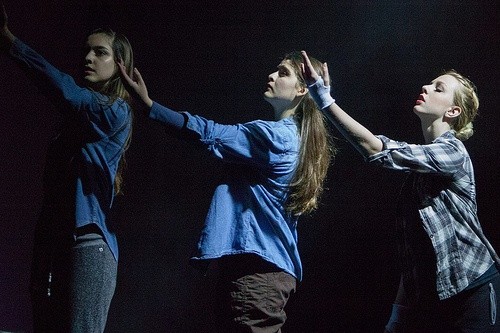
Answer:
[298,50,500,333]
[116,53,329,333]
[0,2,133,333]
